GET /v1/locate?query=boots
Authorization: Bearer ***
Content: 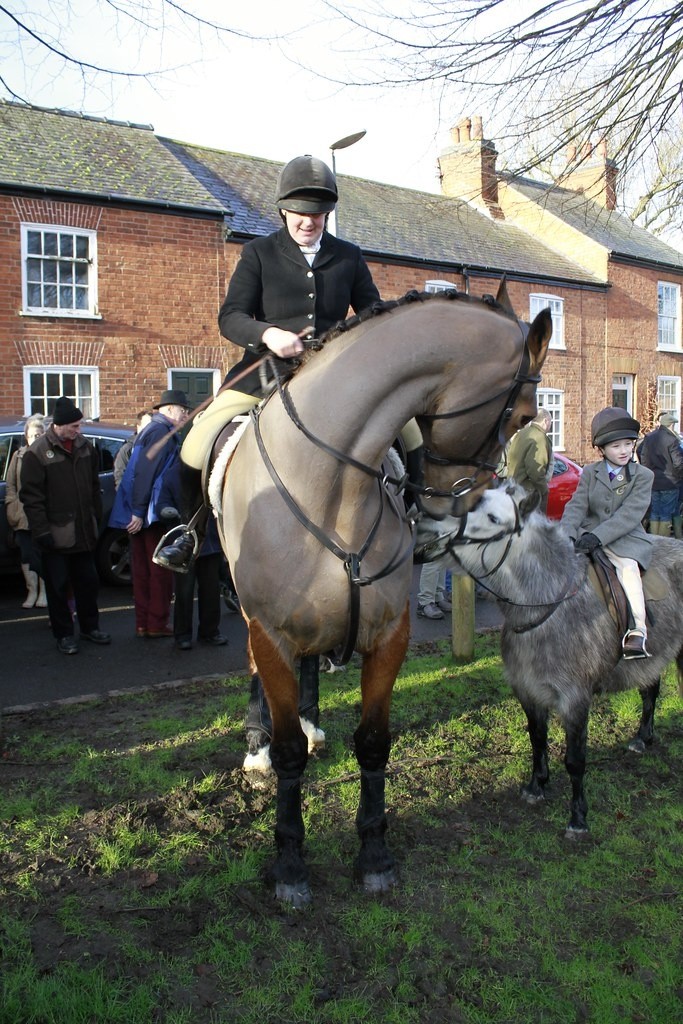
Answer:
[35,574,48,607]
[158,456,202,566]
[673,516,683,537]
[20,564,39,609]
[650,519,670,537]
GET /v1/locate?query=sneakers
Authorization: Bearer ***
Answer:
[57,635,78,655]
[79,627,111,643]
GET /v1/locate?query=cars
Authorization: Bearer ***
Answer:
[545,453,583,519]
[0,416,137,587]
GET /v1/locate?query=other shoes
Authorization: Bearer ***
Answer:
[437,599,454,612]
[623,632,646,655]
[225,596,240,612]
[200,634,228,644]
[136,625,173,638]
[416,602,444,619]
[177,639,191,649]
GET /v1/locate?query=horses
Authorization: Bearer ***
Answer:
[207,273,552,912]
[443,476,683,842]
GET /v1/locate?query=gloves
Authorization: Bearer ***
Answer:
[574,532,599,555]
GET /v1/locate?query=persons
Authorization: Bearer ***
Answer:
[114,411,154,493]
[27,395,110,652]
[108,389,193,636]
[493,408,553,522]
[158,413,228,648]
[416,560,489,619]
[155,156,441,574]
[636,412,683,541]
[5,418,48,608]
[560,407,656,659]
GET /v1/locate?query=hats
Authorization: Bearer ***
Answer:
[661,415,679,426]
[152,392,196,413]
[53,396,83,426]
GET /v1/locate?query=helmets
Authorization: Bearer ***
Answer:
[274,153,339,216]
[590,405,640,448]
